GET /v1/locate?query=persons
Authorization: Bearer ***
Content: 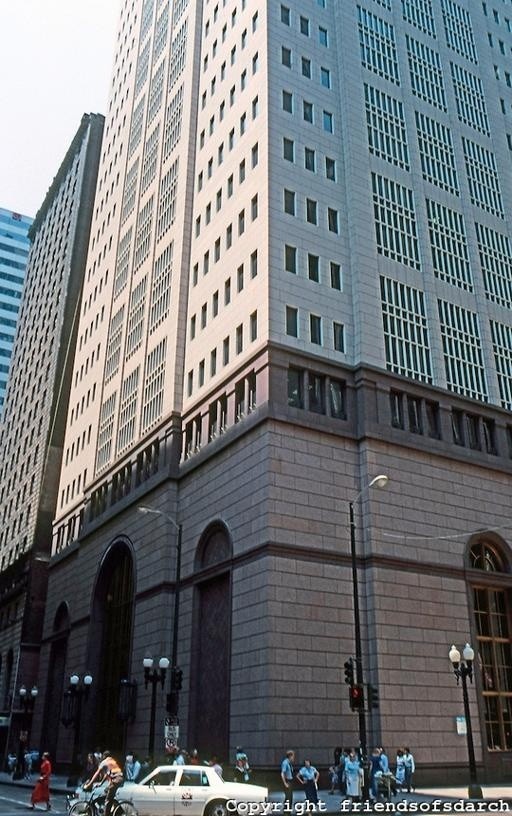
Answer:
[328,746,415,810]
[8,750,39,781]
[280,750,295,816]
[82,745,223,816]
[233,745,251,783]
[296,759,320,815]
[29,751,53,810]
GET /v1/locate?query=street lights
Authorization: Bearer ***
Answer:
[346,472,389,793]
[447,641,485,799]
[11,682,41,781]
[134,503,184,759]
[141,647,170,762]
[64,673,96,787]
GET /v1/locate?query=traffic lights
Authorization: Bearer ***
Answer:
[348,682,365,712]
[343,656,354,686]
[368,682,380,714]
[173,664,185,694]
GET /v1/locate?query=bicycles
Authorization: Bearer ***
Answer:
[66,784,140,816]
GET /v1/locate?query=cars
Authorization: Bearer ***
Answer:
[70,763,271,816]
